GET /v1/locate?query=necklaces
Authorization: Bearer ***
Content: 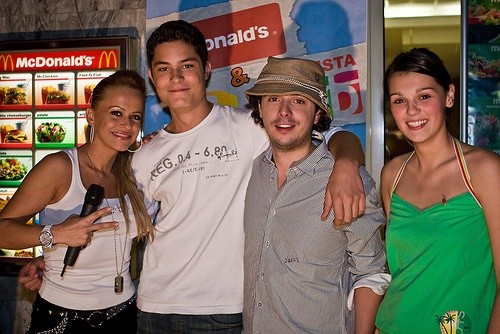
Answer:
[84,142,129,295]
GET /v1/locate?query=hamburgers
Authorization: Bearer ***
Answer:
[47,91,71,104]
[5,88,27,105]
[4,130,27,142]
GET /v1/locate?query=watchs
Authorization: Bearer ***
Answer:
[39,224,54,249]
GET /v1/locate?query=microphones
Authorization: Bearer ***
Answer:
[60,184,104,277]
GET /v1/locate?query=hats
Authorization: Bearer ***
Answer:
[245,56,330,117]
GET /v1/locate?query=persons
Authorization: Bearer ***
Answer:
[19,20,366,334]
[241,56,392,334]
[374,46,500,334]
[0,70,158,334]
